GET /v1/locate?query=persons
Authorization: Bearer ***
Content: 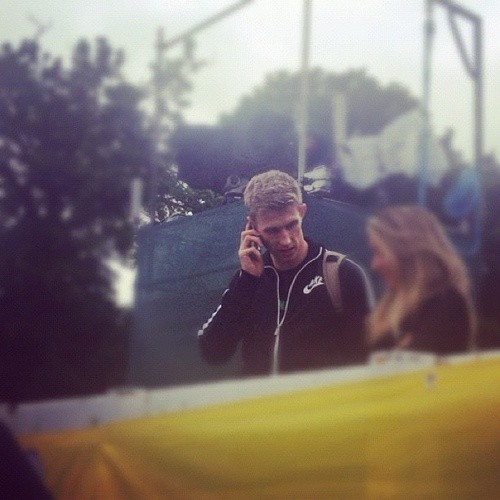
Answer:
[366,127,500,353]
[198,171,375,380]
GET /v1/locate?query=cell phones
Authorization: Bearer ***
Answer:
[250,226,260,252]
